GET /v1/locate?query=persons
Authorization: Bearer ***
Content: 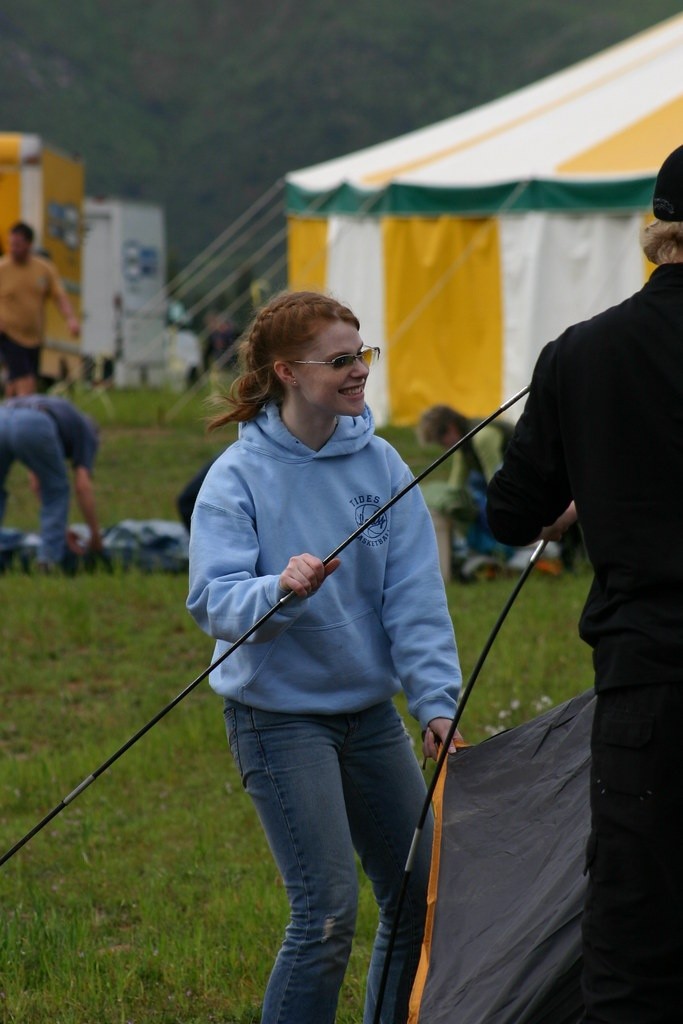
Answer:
[0,223,79,396]
[419,405,561,568]
[166,264,271,389]
[176,454,217,535]
[486,144,683,1023]
[183,290,464,1023]
[0,396,104,578]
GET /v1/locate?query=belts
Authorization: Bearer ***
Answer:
[15,401,49,413]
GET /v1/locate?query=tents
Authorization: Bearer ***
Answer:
[284,12,683,427]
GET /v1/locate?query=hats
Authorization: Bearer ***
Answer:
[653,144,683,222]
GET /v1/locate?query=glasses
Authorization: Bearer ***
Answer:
[294,345,380,370]
